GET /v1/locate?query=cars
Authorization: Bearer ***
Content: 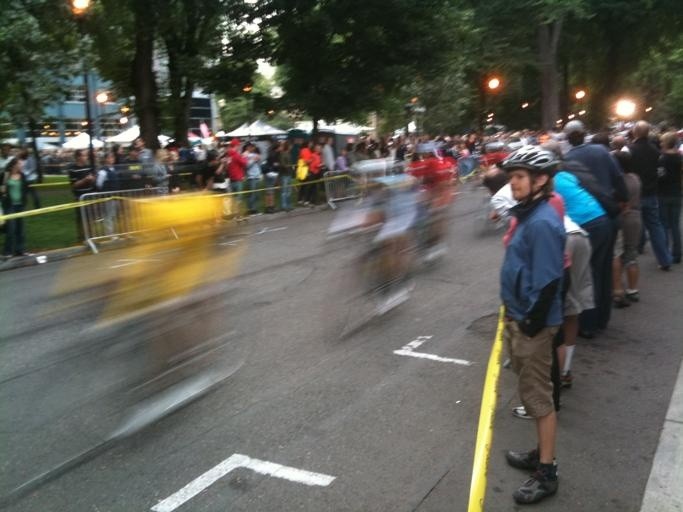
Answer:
[0,136,76,154]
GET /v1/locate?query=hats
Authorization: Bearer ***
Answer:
[563,120,586,138]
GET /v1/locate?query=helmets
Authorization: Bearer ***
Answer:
[496,144,562,175]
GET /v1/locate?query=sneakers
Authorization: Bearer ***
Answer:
[657,264,673,272]
[610,295,632,308]
[626,290,640,303]
[511,401,560,421]
[554,370,574,388]
[669,254,681,264]
[512,468,560,506]
[506,448,556,475]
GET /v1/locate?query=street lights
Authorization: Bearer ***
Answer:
[479,76,500,136]
[405,96,418,134]
[71,0,96,177]
[574,89,586,120]
[241,81,252,146]
[95,91,107,136]
